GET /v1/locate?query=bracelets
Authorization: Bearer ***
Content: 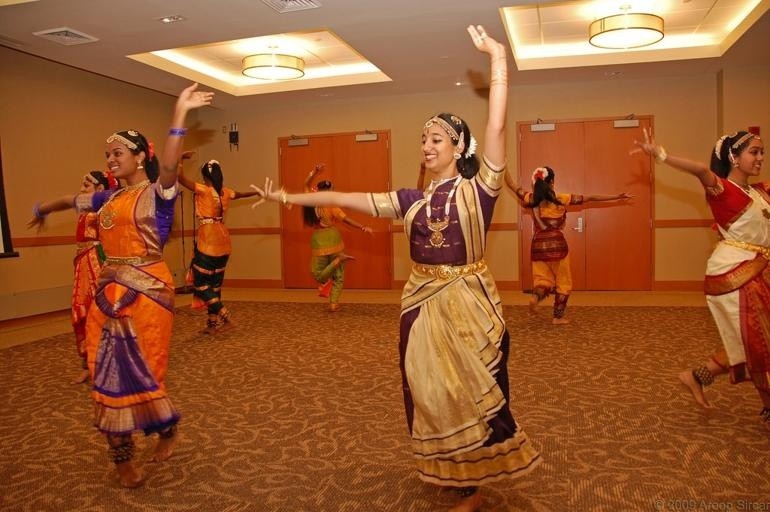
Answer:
[33,203,46,217]
[279,189,291,208]
[656,146,668,161]
[360,224,364,229]
[169,128,184,134]
[487,56,507,83]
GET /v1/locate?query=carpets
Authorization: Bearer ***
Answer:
[1,298,770,510]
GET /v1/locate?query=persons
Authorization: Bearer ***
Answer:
[627,125,770,428]
[251,25,543,511]
[177,148,263,334]
[26,80,214,489]
[303,162,376,311]
[70,168,118,385]
[503,165,636,325]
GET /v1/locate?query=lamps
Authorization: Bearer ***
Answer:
[585,0,665,51]
[240,41,306,85]
